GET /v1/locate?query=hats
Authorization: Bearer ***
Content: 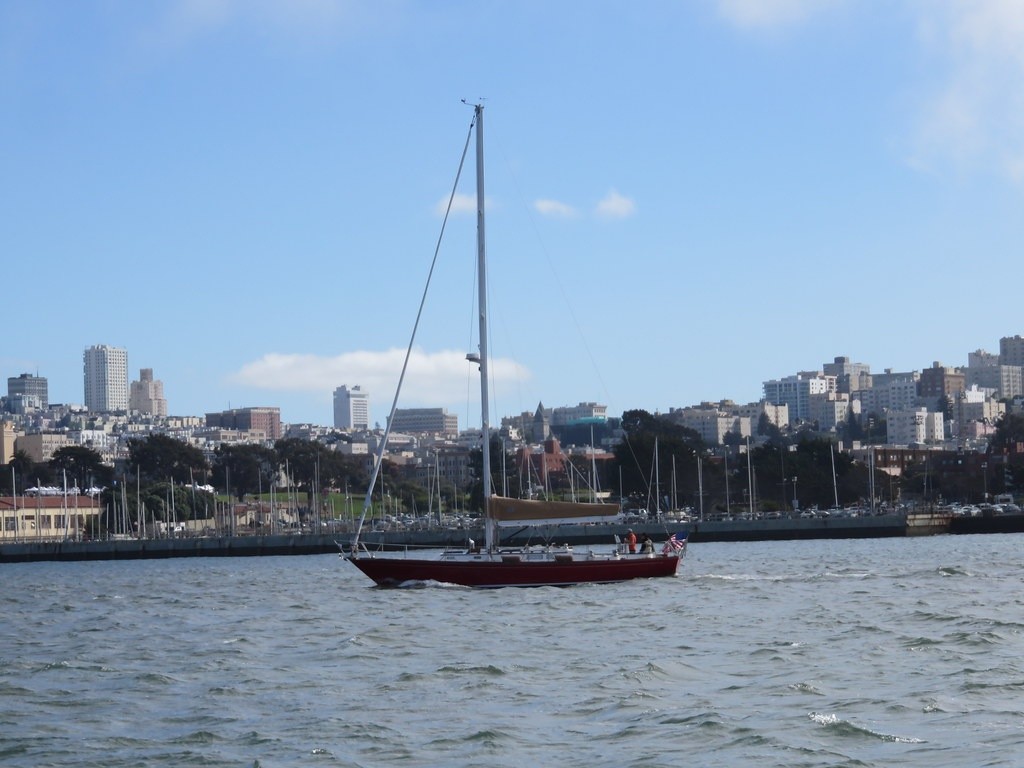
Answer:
[627,528,633,532]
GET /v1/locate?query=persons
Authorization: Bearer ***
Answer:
[624,528,655,554]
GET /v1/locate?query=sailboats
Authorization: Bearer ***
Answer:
[333,98,687,587]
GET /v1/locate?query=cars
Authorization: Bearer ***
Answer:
[308,493,1024,535]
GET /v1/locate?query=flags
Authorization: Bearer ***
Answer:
[662,534,686,553]
